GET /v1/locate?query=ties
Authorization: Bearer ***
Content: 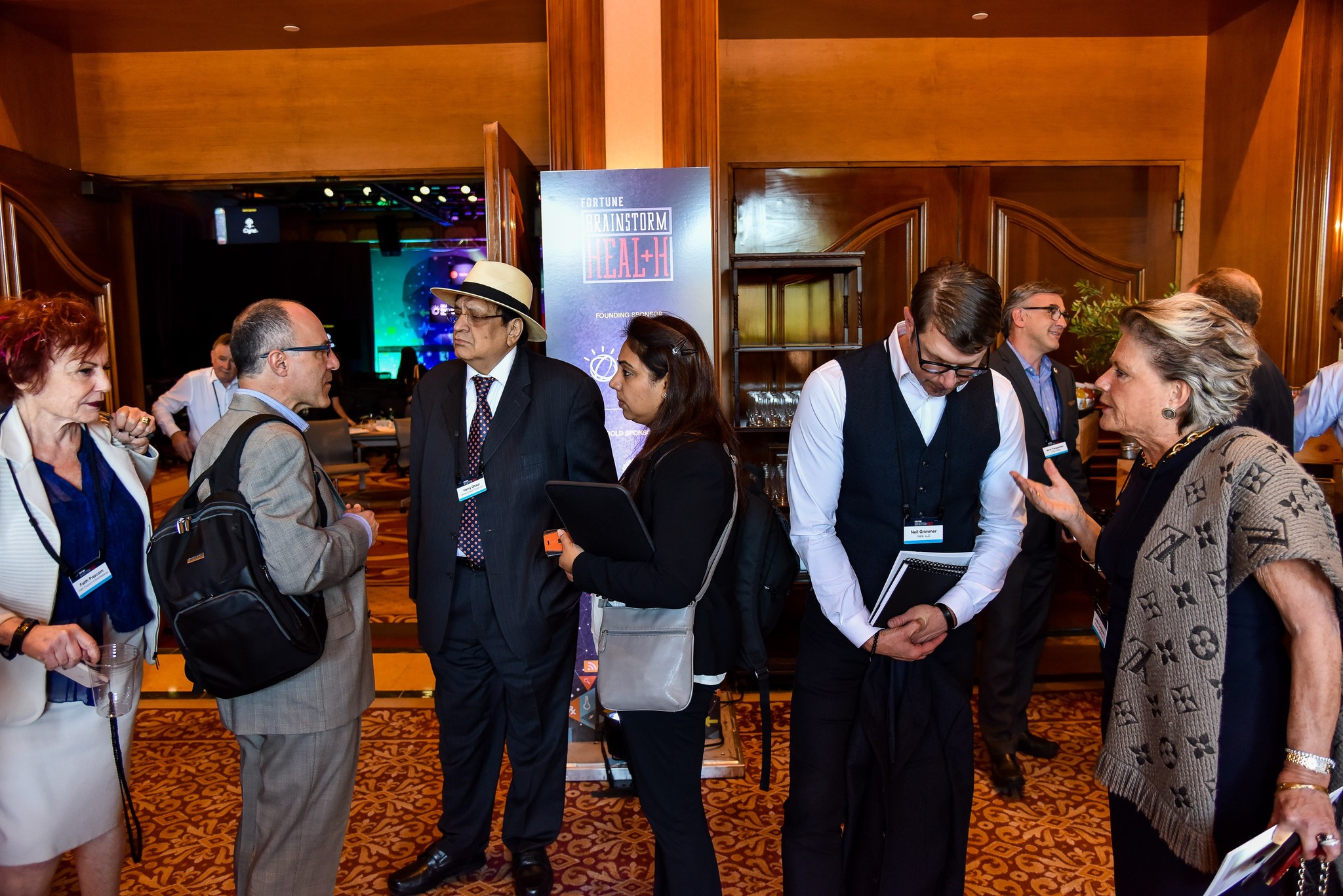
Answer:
[456,375,498,568]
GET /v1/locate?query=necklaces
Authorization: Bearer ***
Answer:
[1140,425,1218,469]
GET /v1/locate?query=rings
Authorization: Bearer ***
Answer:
[1321,839,1340,844]
[139,416,150,426]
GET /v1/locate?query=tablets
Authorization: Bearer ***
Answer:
[543,479,655,564]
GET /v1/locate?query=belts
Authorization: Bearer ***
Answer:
[457,556,486,569]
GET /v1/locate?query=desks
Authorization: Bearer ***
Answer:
[351,420,406,479]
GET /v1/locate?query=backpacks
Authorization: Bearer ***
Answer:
[145,414,328,700]
[640,429,801,680]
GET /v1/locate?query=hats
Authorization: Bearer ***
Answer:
[429,260,548,343]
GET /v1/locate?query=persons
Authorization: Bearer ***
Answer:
[781,260,1343,895]
[332,369,397,473]
[151,332,241,483]
[559,316,743,896]
[0,292,160,896]
[398,346,423,418]
[386,260,618,894]
[188,299,380,895]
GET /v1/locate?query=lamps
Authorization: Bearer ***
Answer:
[324,181,477,222]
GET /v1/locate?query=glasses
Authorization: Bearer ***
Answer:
[445,307,502,325]
[260,341,331,359]
[1022,306,1070,322]
[914,323,991,377]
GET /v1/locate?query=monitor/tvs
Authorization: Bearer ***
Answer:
[212,203,281,247]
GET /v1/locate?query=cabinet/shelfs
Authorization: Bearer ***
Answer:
[730,251,866,791]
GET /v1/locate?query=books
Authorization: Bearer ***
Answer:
[869,549,975,627]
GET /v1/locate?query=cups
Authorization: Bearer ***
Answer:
[746,391,802,427]
[762,463,788,505]
[83,643,140,718]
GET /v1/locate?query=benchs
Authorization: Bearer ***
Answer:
[303,419,370,489]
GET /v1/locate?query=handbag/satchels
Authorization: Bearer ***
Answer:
[597,601,696,712]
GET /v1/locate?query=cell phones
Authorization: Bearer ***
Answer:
[1262,842,1302,887]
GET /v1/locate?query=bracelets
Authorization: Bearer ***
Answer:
[1285,747,1336,776]
[1276,783,1331,795]
[10,617,41,656]
[1080,548,1096,563]
[933,603,955,632]
[867,633,878,661]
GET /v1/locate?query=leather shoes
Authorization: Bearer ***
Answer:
[992,752,1026,789]
[512,846,554,896]
[387,836,487,896]
[1016,730,1059,757]
